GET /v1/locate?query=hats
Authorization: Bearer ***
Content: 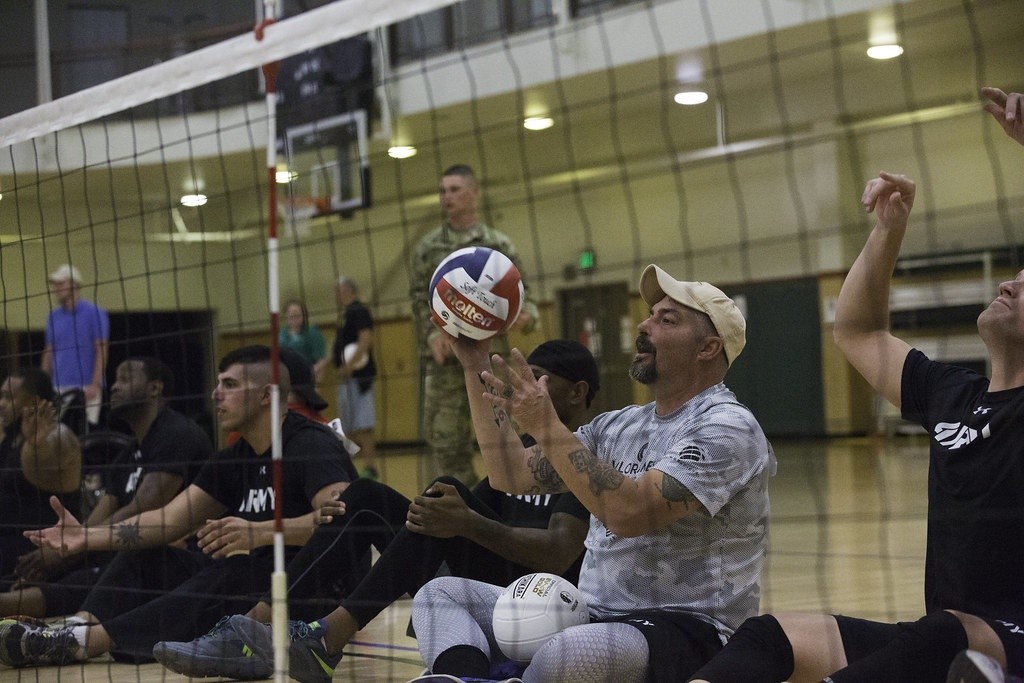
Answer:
[527,340,600,397]
[640,264,746,370]
[50,265,83,286]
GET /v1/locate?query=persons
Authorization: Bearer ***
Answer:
[155,338,599,683]
[314,278,380,481]
[688,85,1024,683]
[410,263,772,683]
[0,344,357,683]
[409,164,539,489]
[276,301,325,388]
[40,264,110,399]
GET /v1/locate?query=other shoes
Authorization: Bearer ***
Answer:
[360,465,379,481]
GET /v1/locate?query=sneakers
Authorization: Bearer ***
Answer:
[230,614,343,683]
[153,614,274,679]
[0,615,99,666]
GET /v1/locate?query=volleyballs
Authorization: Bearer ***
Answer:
[492,573,591,667]
[427,245,527,342]
[342,343,369,370]
